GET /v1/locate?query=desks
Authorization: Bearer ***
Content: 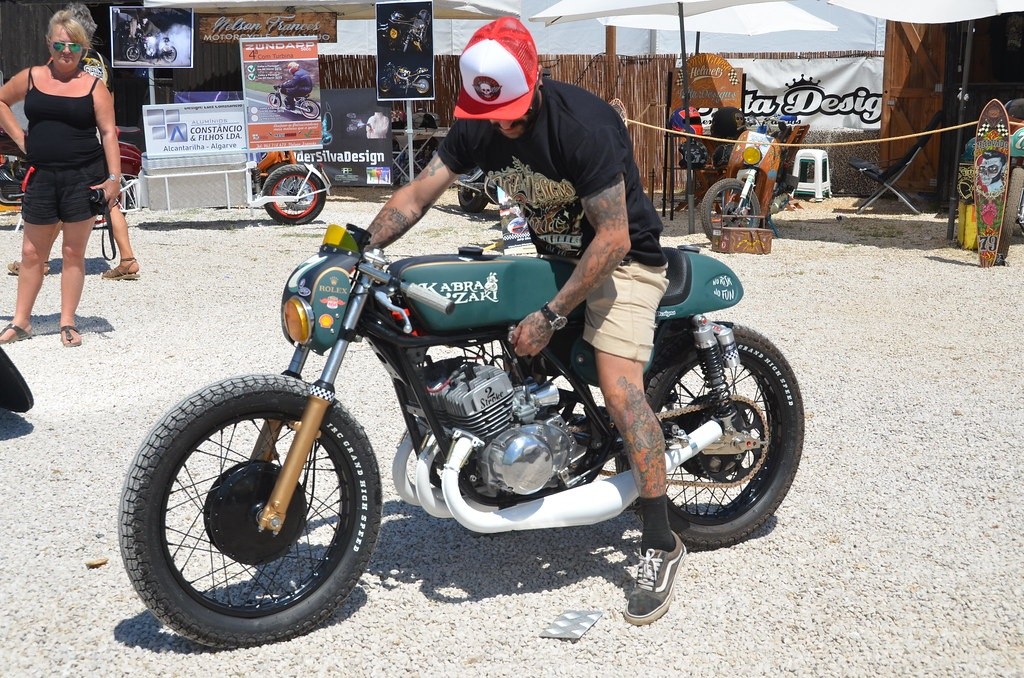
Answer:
[391,126,451,183]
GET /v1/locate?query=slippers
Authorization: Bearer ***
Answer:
[0,323,31,344]
[62,325,81,348]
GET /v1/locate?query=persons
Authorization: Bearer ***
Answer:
[136,16,164,63]
[274,62,313,110]
[7,1,141,281]
[360,16,687,625]
[0,10,123,347]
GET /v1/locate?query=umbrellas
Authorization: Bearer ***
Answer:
[528,0,1024,37]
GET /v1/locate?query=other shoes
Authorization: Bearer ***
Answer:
[285,105,295,110]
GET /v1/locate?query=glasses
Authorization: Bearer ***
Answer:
[48,38,83,53]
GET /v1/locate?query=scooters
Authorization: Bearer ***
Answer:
[699,113,800,243]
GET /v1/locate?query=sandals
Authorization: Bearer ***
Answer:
[101,257,140,280]
[8,259,50,276]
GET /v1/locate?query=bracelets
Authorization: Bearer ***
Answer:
[109,174,121,183]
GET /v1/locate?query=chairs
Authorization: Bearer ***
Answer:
[113,125,141,215]
[847,110,942,213]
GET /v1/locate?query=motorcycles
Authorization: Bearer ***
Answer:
[378,7,431,54]
[115,219,805,646]
[267,79,321,120]
[378,58,432,97]
[250,150,332,223]
[125,32,177,64]
[0,126,145,220]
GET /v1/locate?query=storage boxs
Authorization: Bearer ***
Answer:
[712,213,772,254]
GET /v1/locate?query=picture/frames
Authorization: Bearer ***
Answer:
[374,0,435,101]
[109,6,194,69]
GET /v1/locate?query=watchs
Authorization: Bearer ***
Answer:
[540,301,567,331]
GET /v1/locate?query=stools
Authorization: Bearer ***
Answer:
[790,148,832,201]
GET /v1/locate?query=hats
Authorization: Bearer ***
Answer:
[454,16,538,119]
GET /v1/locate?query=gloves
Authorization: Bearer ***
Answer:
[273,85,281,90]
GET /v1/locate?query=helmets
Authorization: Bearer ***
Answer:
[669,106,703,136]
[287,62,299,74]
[697,106,718,136]
[709,106,746,137]
[679,140,708,169]
[711,143,735,171]
[142,17,148,24]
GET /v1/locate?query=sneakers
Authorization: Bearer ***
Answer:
[624,530,687,625]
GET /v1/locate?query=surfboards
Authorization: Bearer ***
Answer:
[973,97,1012,268]
